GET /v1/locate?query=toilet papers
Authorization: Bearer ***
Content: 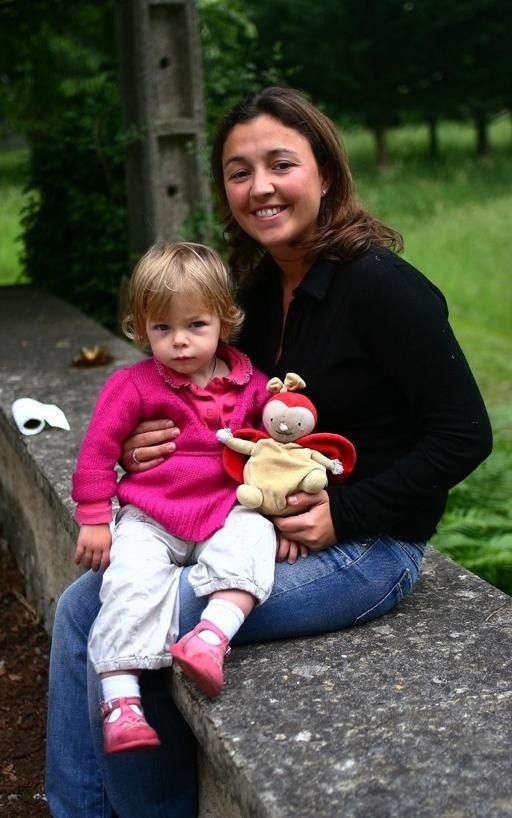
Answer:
[12,397,70,436]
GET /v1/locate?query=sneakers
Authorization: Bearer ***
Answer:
[99,697,163,755]
[170,621,230,699]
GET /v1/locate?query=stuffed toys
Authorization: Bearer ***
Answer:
[217,372,357,515]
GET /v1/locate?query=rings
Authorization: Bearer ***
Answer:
[130,447,140,464]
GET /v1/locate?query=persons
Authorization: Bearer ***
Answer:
[74,231,308,752]
[46,88,494,818]
